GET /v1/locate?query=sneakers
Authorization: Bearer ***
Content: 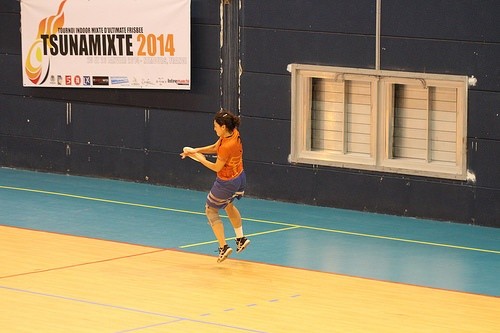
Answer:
[235,236,250,253]
[217,244,232,263]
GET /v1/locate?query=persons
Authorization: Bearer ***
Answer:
[180,108,251,262]
